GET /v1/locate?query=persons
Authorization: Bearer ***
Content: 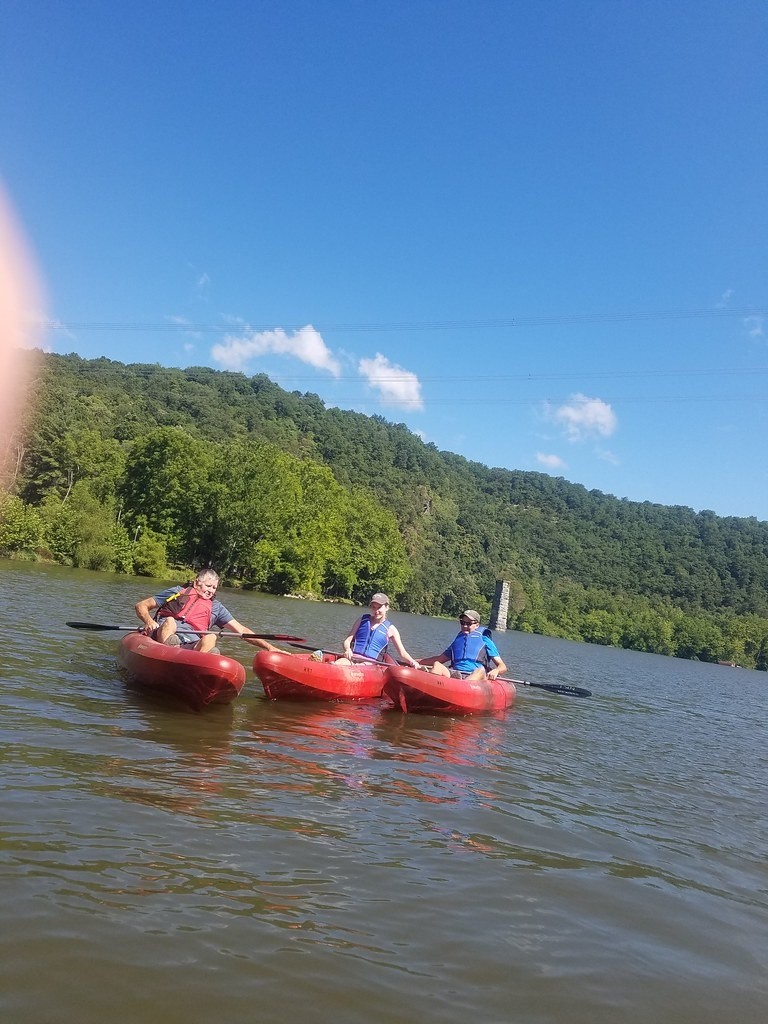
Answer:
[416,610,507,680]
[308,593,422,670]
[135,569,292,655]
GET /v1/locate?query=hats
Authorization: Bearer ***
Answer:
[459,610,480,623]
[370,592,390,605]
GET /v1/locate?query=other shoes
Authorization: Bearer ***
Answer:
[307,650,323,663]
[420,666,429,672]
[450,670,462,680]
[163,634,180,648]
[208,647,220,654]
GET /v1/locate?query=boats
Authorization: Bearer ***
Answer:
[252,651,388,701]
[383,664,517,716]
[118,632,246,707]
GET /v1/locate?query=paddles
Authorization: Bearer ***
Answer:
[64,619,310,644]
[284,640,398,668]
[423,663,595,700]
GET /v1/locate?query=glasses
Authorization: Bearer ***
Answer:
[460,620,476,626]
